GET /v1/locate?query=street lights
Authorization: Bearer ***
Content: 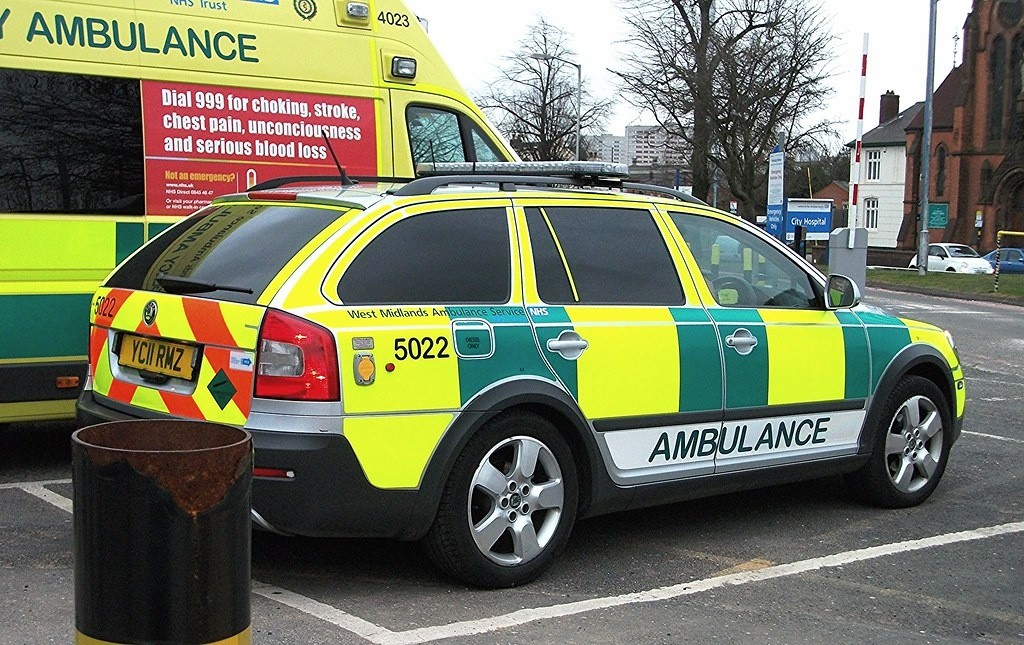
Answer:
[530,53,581,163]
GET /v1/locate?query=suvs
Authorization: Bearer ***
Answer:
[72,160,968,592]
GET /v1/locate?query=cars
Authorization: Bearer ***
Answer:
[982,246,1024,275]
[908,243,995,275]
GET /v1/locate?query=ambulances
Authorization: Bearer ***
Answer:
[0,0,525,419]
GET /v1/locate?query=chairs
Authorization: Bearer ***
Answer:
[636,250,680,303]
